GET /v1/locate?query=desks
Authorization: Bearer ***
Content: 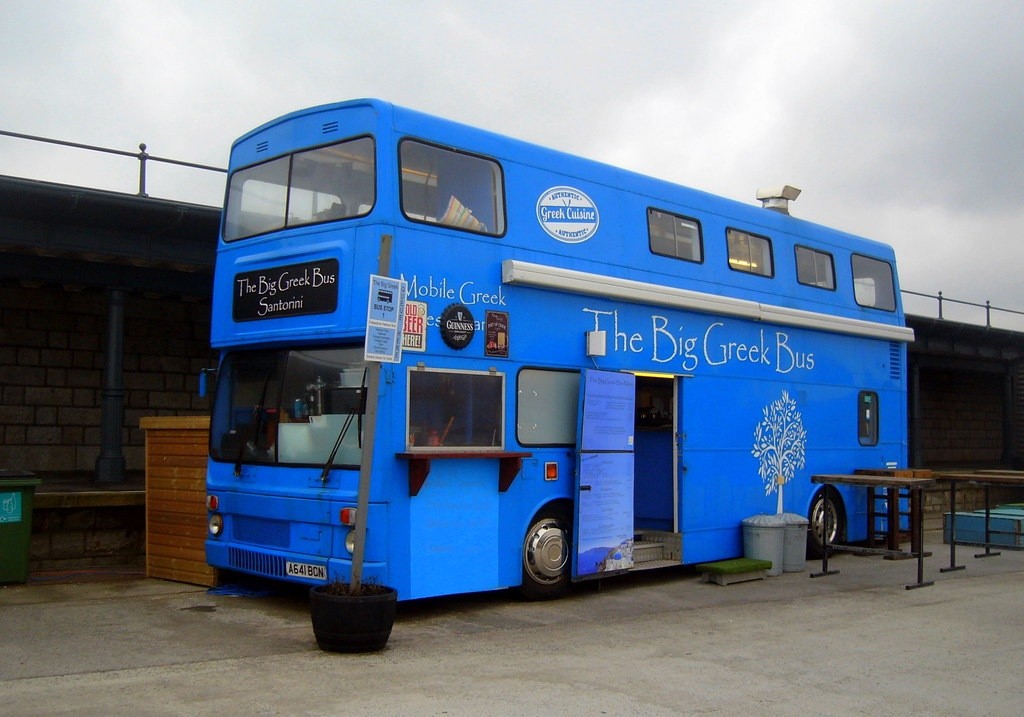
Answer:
[810,469,1024,589]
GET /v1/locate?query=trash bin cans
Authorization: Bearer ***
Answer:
[0,479,42,585]
[780,513,809,572]
[743,515,786,576]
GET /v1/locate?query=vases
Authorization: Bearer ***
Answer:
[310,584,399,652]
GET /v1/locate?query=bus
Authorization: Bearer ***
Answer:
[198,97,915,603]
